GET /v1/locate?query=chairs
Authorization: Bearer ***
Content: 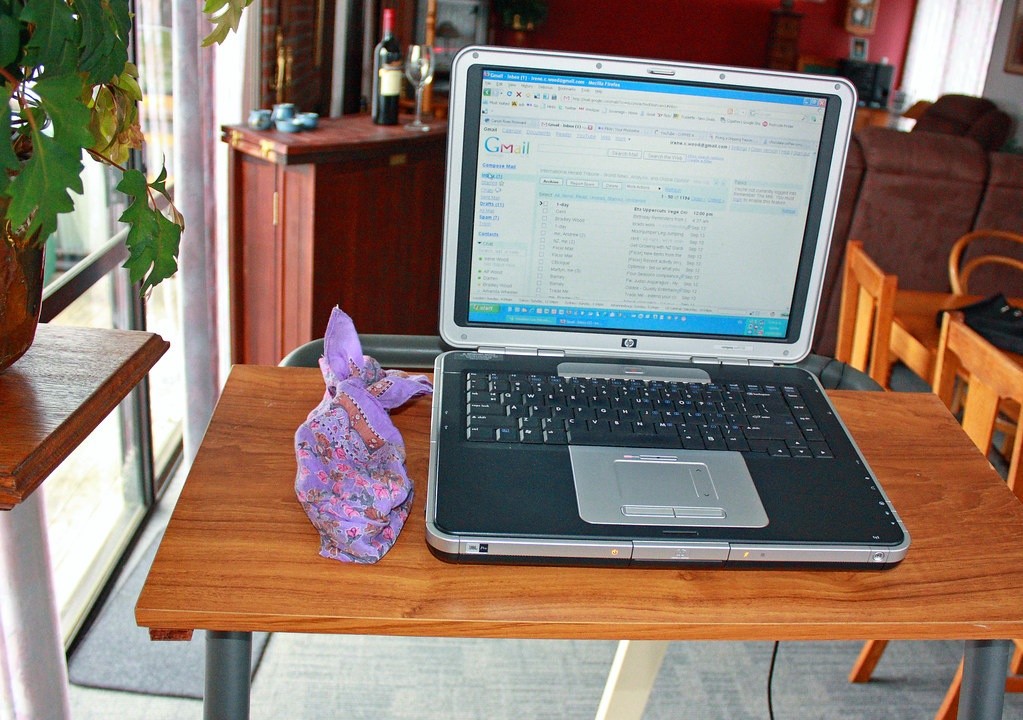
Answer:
[836,238,931,393]
[934,307,1023,504]
[948,228,1023,295]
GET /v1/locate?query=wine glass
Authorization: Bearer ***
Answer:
[404,44,435,132]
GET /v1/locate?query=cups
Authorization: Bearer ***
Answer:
[274,118,304,133]
[295,112,319,129]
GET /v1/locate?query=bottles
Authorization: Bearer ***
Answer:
[369,7,403,126]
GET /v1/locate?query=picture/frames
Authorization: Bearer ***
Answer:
[1003,0,1023,76]
[848,36,869,62]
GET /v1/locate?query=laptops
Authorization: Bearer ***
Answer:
[424,45,911,566]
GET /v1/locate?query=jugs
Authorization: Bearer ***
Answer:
[248,109,272,130]
[271,103,295,123]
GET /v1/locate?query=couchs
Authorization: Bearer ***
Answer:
[810,125,1021,355]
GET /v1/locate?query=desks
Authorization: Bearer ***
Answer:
[0,321,172,720]
[884,290,1023,461]
[135,362,1023,719]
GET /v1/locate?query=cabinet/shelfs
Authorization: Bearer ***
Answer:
[219,108,449,368]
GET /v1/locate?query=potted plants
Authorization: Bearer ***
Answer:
[0,0,185,375]
